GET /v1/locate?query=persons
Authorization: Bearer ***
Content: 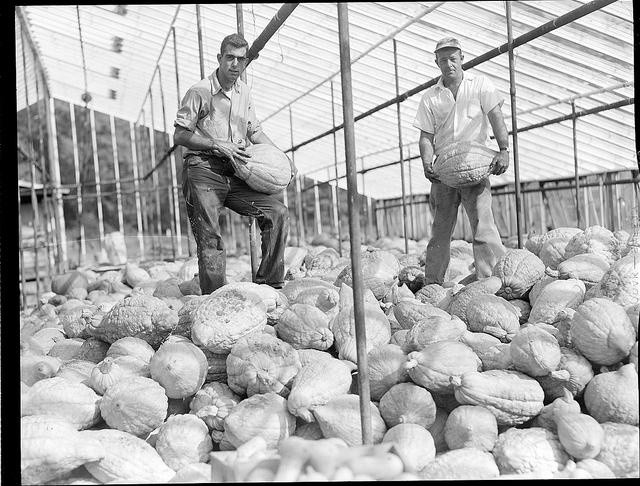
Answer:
[411,34,511,286]
[173,32,300,295]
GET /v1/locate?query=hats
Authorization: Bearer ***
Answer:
[434,38,461,53]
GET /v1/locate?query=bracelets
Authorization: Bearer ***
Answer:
[499,146,511,153]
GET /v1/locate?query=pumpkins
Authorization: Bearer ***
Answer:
[20,226,640,485]
[228,143,292,195]
[431,144,499,189]
